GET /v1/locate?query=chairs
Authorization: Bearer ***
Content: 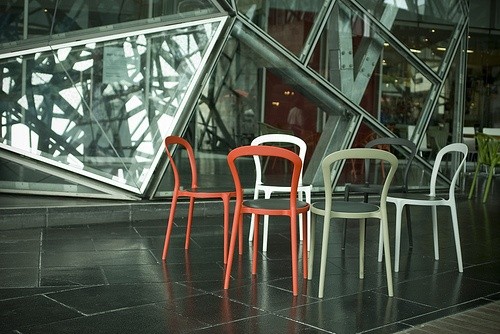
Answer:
[468,132,500,205]
[158,132,246,266]
[306,146,398,296]
[339,136,418,257]
[220,144,312,297]
[374,142,471,275]
[250,133,315,256]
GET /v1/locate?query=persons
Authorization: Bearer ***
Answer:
[286,102,305,132]
